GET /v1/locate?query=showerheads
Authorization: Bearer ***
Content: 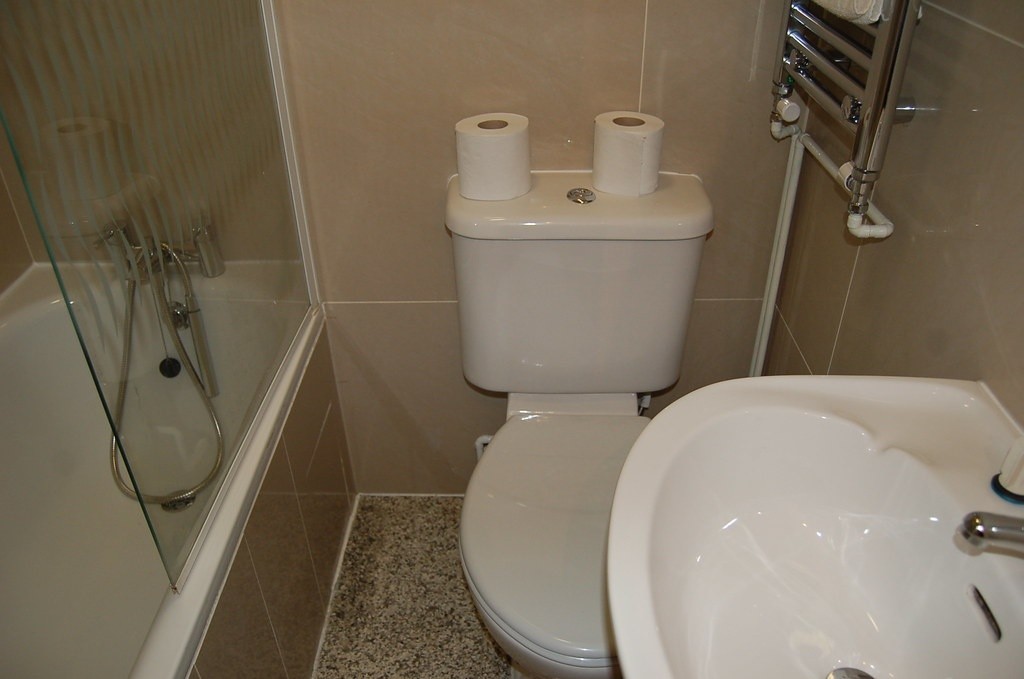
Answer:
[179,281,221,396]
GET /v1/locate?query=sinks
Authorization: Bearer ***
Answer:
[604,372,1024,679]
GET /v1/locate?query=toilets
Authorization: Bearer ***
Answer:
[441,166,715,676]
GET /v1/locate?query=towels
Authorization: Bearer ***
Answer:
[813,0,923,27]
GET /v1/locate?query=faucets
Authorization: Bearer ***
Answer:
[955,509,1022,559]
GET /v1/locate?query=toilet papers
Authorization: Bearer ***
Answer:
[454,110,533,198]
[592,111,665,197]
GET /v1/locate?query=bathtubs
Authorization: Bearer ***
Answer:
[0,261,361,679]
